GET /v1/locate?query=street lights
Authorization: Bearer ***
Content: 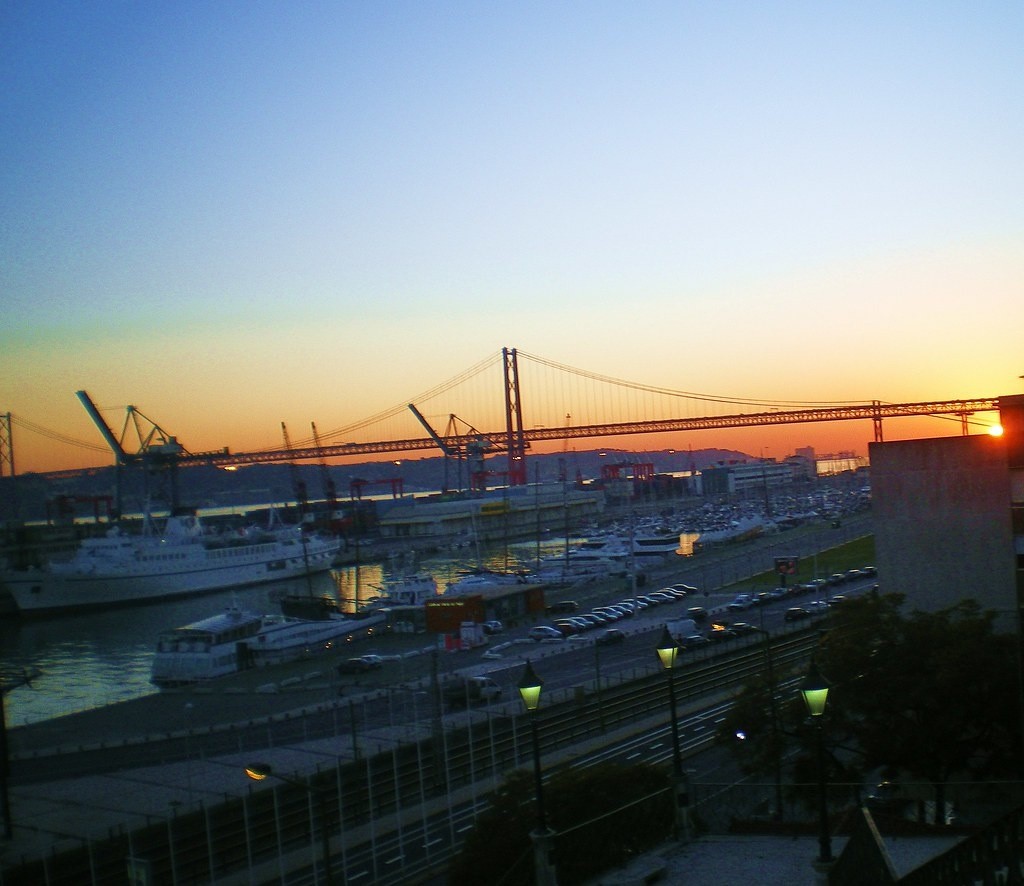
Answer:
[798,662,838,866]
[244,760,334,886]
[517,657,557,886]
[656,625,692,842]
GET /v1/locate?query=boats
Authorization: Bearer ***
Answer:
[149,605,389,688]
[1,508,344,619]
[281,532,705,626]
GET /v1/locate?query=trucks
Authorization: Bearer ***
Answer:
[443,676,503,708]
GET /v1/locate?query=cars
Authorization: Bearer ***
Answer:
[596,628,625,645]
[713,565,881,654]
[682,634,709,650]
[529,581,700,641]
[339,658,372,673]
[362,656,383,667]
